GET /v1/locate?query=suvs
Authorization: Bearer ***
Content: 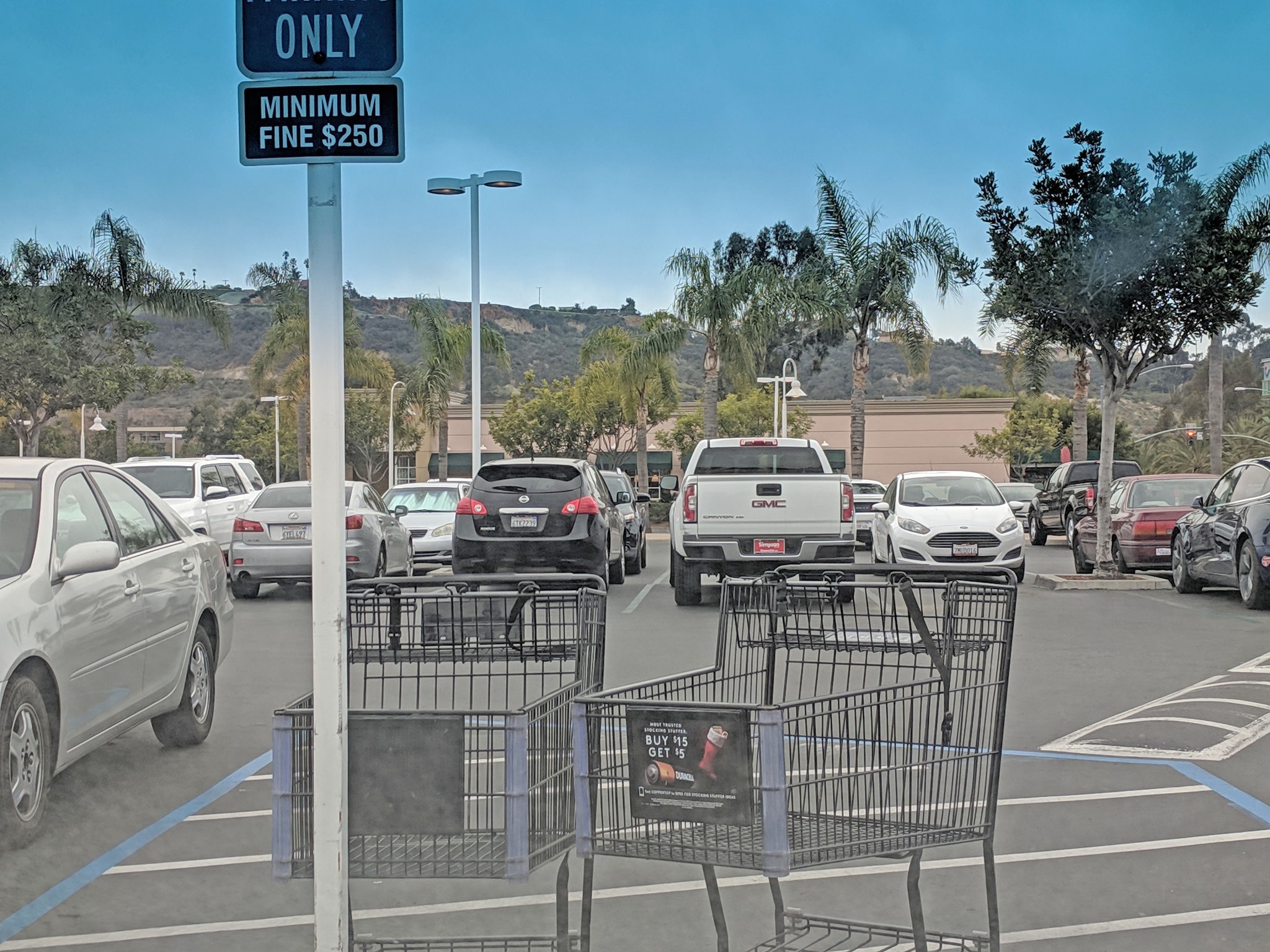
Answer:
[851,479,887,548]
[107,455,265,573]
[597,466,650,574]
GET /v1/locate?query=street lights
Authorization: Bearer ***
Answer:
[426,170,525,482]
[259,395,293,485]
[9,419,31,458]
[164,433,184,458]
[756,357,807,438]
[81,401,106,457]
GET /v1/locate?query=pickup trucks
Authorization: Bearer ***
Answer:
[659,437,856,604]
[1028,460,1142,549]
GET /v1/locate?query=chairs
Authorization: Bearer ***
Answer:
[904,486,925,504]
[948,486,980,504]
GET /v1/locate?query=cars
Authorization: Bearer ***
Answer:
[994,482,1046,532]
[382,483,470,566]
[869,472,1026,583]
[451,442,632,595]
[1170,456,1270,610]
[228,480,416,598]
[1072,473,1220,575]
[1,453,234,850]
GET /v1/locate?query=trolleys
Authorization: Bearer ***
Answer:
[268,562,1018,950]
